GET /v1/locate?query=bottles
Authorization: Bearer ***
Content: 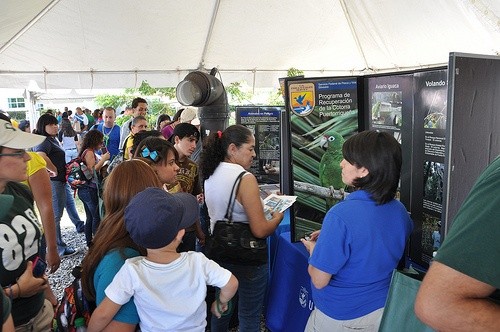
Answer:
[80,162,93,180]
[74,318,87,332]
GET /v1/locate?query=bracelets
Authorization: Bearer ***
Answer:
[215,290,232,315]
[15,278,21,298]
[3,284,13,303]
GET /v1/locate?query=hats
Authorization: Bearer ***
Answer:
[180,108,197,124]
[19,120,30,130]
[0,113,46,150]
[124,187,199,249]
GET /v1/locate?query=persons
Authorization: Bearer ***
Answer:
[0,97,204,332]
[85,186,239,332]
[198,124,284,332]
[299,130,415,332]
[412,154,500,332]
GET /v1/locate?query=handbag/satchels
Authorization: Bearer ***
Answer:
[208,171,268,266]
[107,135,131,173]
[51,267,92,332]
[66,150,96,198]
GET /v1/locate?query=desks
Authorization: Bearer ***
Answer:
[264,231,314,332]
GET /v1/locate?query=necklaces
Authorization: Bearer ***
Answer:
[102,123,115,136]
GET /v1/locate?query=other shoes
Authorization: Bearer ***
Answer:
[87,240,94,247]
[77,225,86,234]
[60,243,77,260]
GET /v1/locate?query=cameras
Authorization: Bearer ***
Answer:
[31,256,47,278]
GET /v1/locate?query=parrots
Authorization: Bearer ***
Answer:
[320,131,349,212]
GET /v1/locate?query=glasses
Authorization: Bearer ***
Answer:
[0,150,25,158]
